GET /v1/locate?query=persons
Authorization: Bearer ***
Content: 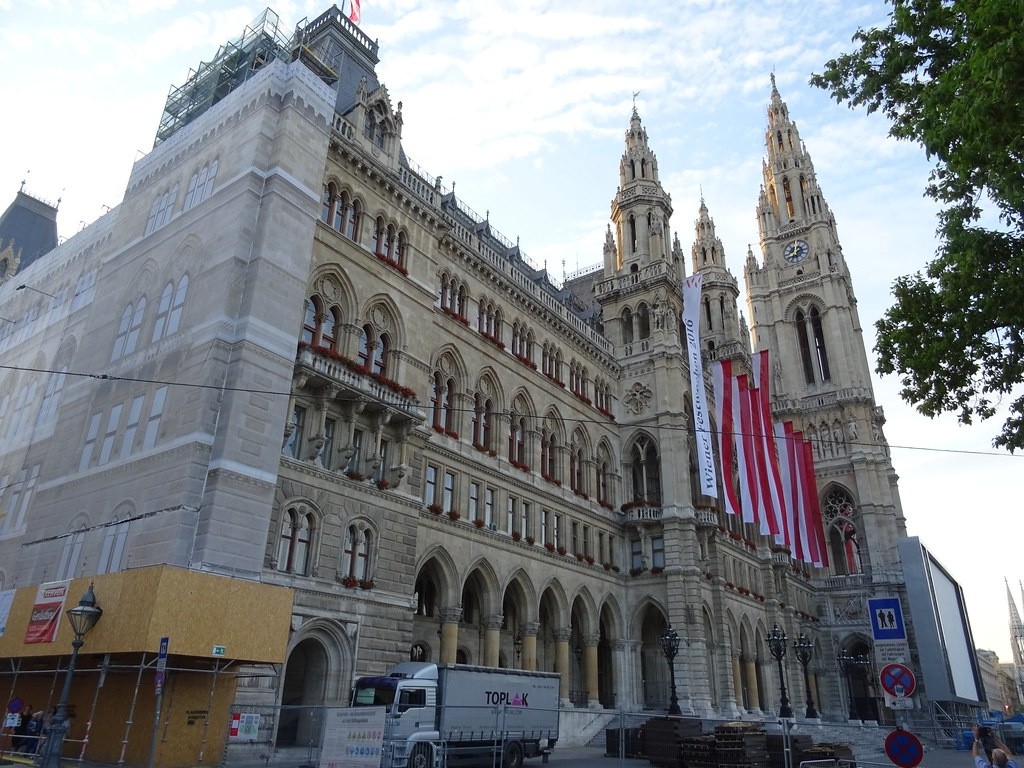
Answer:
[25,710,44,759]
[10,705,32,757]
[972,722,1018,768]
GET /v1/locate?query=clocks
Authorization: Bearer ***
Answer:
[783,239,809,264]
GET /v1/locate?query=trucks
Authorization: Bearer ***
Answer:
[349,662,562,768]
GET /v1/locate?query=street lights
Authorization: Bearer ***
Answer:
[792,632,820,718]
[660,626,683,716]
[855,647,875,719]
[35,580,103,768]
[835,647,860,721]
[766,623,794,718]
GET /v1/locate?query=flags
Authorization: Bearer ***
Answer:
[349,0,360,27]
